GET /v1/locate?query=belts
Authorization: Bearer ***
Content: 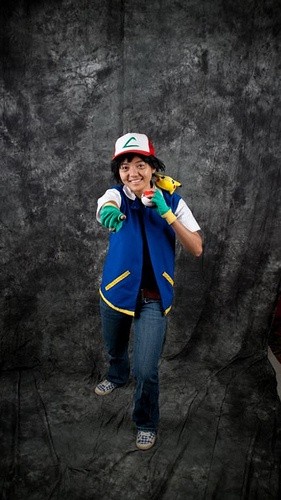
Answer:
[138,289,161,299]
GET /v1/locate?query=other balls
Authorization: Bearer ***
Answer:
[141,191,155,206]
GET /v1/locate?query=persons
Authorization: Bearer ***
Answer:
[92,130,204,450]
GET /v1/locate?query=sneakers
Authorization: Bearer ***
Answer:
[95,379,119,396]
[136,429,157,449]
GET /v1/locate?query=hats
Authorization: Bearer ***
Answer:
[111,133,155,161]
